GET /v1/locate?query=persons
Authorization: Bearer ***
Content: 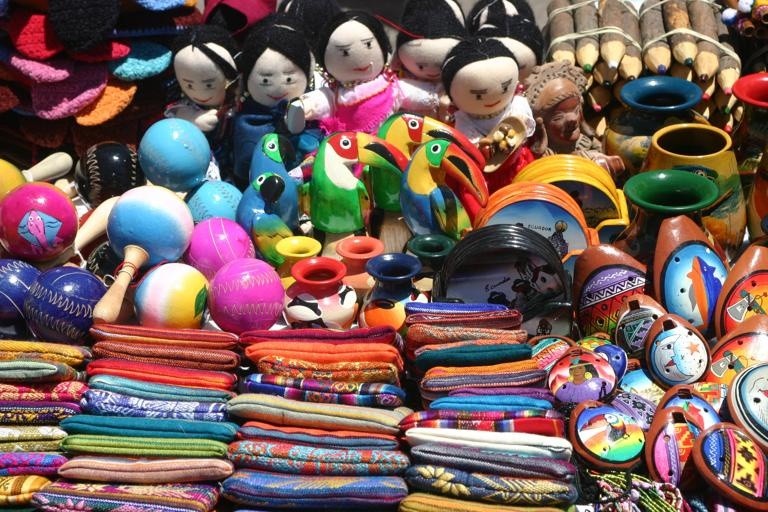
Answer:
[162,2,624,192]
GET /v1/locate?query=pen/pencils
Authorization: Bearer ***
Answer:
[548,1,743,138]
